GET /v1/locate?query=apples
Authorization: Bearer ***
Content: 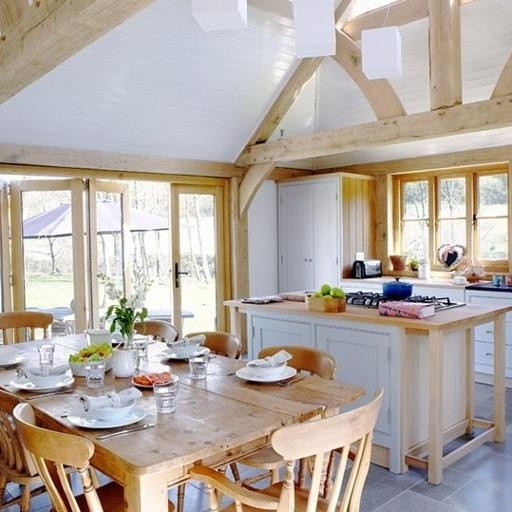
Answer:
[311,283,344,299]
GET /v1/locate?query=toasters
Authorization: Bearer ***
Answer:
[353,260,383,279]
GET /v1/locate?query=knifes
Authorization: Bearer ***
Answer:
[96,423,156,440]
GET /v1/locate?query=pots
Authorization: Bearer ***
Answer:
[383,276,412,298]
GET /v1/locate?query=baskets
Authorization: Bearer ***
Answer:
[306,295,345,312]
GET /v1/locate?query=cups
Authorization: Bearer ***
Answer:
[84,360,105,389]
[133,336,147,356]
[113,348,136,378]
[153,381,176,413]
[37,344,54,365]
[189,353,207,380]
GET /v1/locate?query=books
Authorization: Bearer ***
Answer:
[379,299,436,319]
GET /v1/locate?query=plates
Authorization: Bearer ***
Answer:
[161,347,211,359]
[10,376,74,393]
[132,373,179,388]
[67,406,146,429]
[236,365,297,383]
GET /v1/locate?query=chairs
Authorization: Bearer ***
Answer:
[1,390,99,511]
[1,311,53,346]
[215,346,339,501]
[184,331,242,359]
[11,401,177,512]
[189,385,384,511]
[133,320,179,343]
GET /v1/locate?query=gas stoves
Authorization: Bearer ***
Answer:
[345,290,466,311]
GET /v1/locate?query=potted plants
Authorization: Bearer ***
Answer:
[97,267,148,378]
[409,256,422,271]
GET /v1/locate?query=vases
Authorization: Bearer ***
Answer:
[390,256,408,271]
[111,347,139,378]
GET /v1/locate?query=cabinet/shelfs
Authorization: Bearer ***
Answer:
[341,279,465,302]
[247,311,475,474]
[465,290,512,388]
[275,172,376,293]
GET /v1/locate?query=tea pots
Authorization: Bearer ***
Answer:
[86,330,122,351]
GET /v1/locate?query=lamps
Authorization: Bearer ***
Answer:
[191,0,402,81]
